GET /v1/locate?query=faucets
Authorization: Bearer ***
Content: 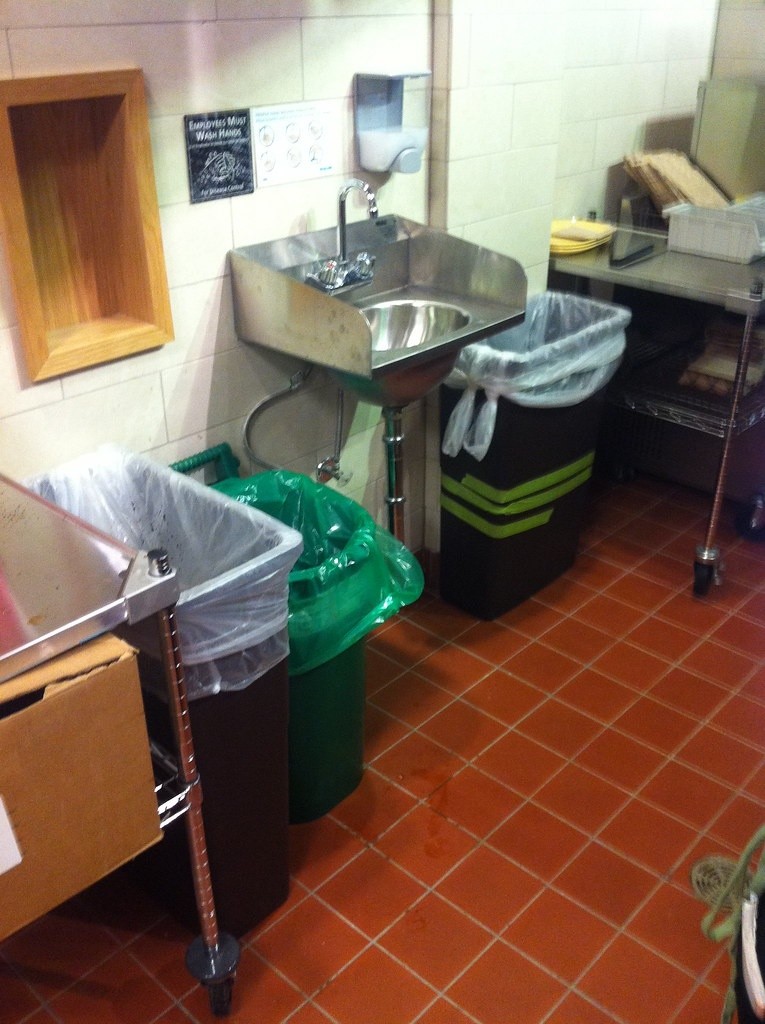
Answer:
[306,179,379,295]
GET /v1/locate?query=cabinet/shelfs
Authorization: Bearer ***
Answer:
[550,217,765,598]
[0,473,241,1016]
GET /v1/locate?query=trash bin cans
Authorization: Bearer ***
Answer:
[205,471,380,830]
[24,450,305,944]
[435,287,630,623]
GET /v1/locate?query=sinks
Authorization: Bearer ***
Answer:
[230,213,529,408]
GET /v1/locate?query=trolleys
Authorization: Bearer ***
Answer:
[545,219,765,597]
[1,467,242,1017]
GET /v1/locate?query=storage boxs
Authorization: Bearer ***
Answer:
[663,204,765,265]
[0,632,167,942]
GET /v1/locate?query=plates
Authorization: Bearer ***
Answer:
[550,219,616,253]
